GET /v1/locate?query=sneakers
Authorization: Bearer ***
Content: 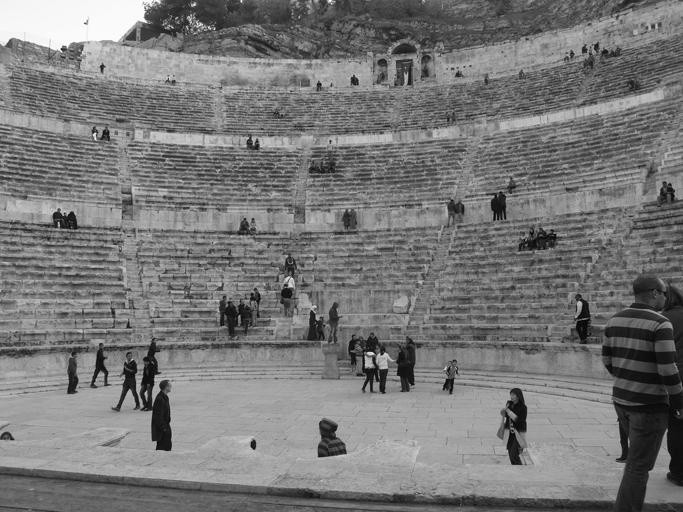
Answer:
[110,406,154,412]
[615,454,629,464]
[666,471,683,489]
[67,383,111,395]
[361,383,415,394]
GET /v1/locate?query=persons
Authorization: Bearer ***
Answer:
[51,41,683,512]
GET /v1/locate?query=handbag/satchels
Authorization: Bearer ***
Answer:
[496,400,511,440]
